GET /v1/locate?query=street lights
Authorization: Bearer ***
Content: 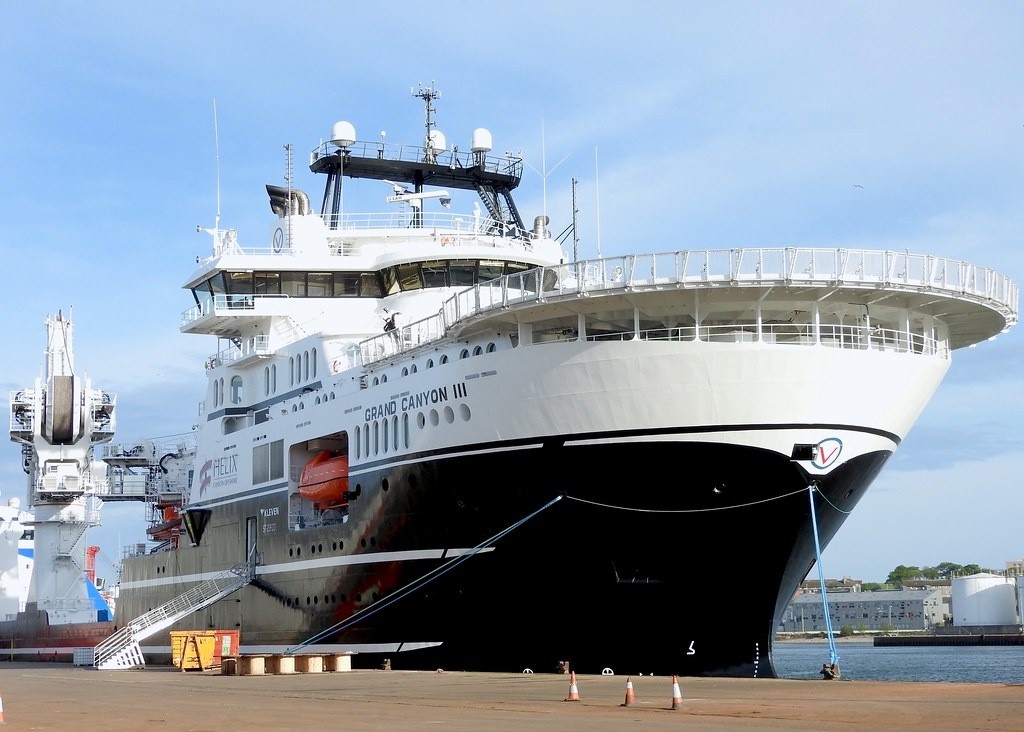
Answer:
[800,607,804,632]
[889,606,892,630]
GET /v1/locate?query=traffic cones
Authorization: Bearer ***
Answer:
[564,671,581,700]
[621,677,638,707]
[672,674,683,710]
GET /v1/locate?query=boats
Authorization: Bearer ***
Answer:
[112,79,1021,679]
